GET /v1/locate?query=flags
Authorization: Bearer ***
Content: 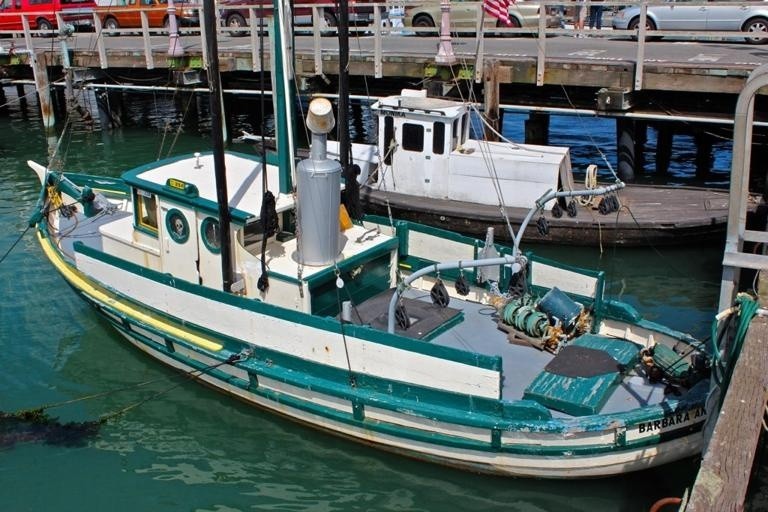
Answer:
[482,0,515,28]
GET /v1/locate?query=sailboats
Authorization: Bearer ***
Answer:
[24,0,723,479]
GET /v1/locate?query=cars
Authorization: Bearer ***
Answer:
[0,0,97,37]
[221,1,391,37]
[402,1,562,39]
[611,0,767,44]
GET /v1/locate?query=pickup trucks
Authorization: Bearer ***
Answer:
[95,0,202,37]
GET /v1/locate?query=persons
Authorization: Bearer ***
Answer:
[573,0,589,39]
[588,0,604,38]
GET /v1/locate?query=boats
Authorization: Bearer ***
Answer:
[242,90,763,246]
